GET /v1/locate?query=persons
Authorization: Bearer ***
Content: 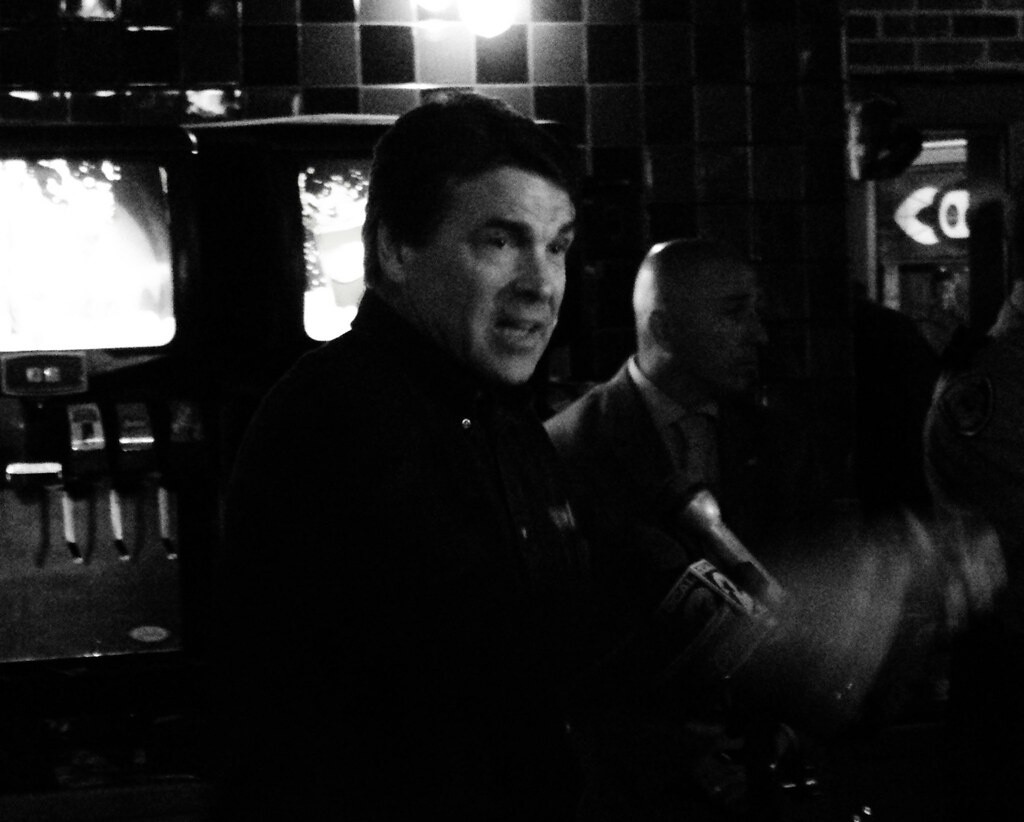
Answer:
[203,94,747,822]
[541,240,768,564]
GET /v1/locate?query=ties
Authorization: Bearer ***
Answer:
[676,414,715,485]
